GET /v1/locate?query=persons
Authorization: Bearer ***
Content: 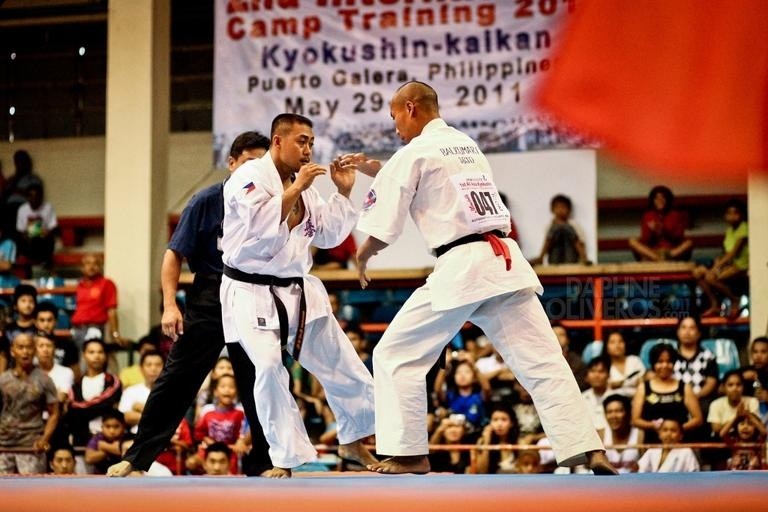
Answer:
[337,81,620,475]
[498,191,519,243]
[1,148,58,279]
[106,130,290,476]
[696,205,749,316]
[630,185,693,261]
[0,227,767,476]
[533,194,587,265]
[219,112,379,475]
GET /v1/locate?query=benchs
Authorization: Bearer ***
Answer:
[311,193,749,341]
[52,192,181,366]
